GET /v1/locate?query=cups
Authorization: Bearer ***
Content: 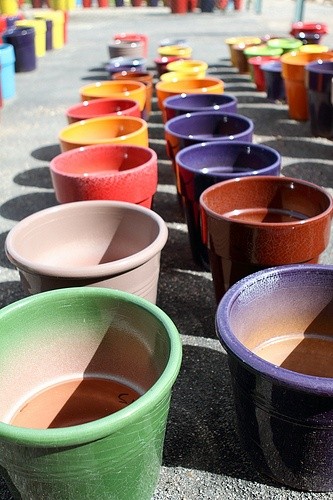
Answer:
[67,22,333,123]
[199,174,333,292]
[0,286,184,500]
[163,112,255,173]
[174,140,281,262]
[215,263,333,493]
[82,0,243,13]
[0,0,74,109]
[4,199,169,307]
[49,143,158,210]
[57,118,149,152]
